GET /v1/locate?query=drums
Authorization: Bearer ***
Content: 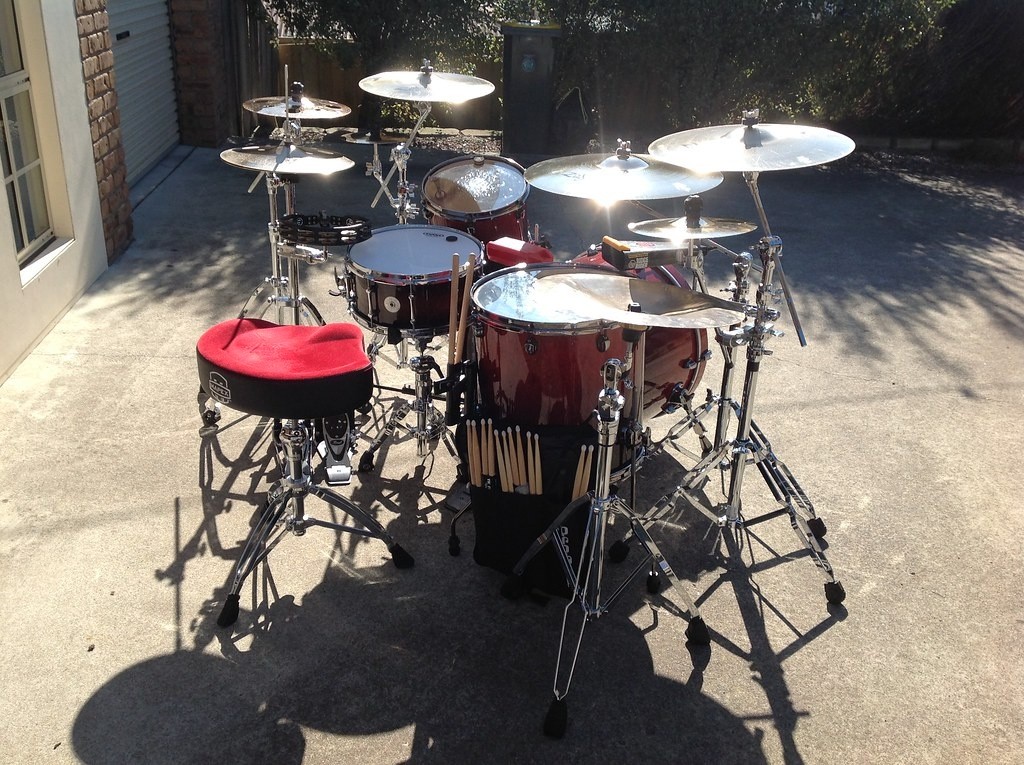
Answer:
[346,224,484,337]
[421,154,531,262]
[470,262,646,489]
[564,242,708,420]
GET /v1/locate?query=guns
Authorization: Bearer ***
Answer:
[242,96,352,119]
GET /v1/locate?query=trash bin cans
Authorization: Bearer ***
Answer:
[498,17,564,170]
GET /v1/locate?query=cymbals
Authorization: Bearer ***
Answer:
[534,273,745,329]
[628,217,758,241]
[648,123,856,172]
[279,210,371,247]
[358,71,496,102]
[525,140,724,200]
[220,147,355,175]
